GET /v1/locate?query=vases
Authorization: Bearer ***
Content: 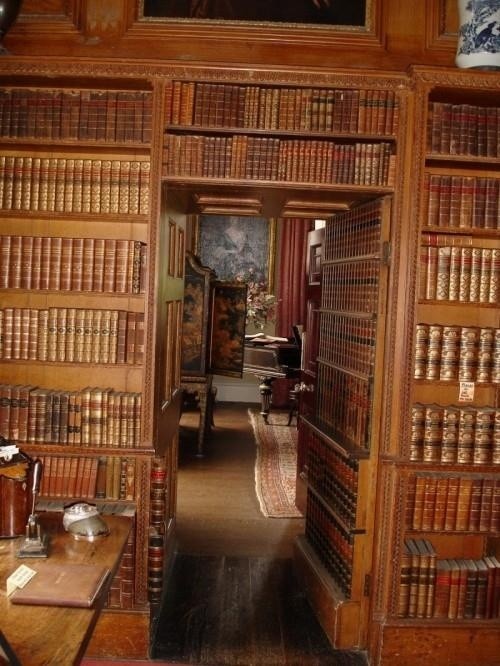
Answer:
[456,0,500,68]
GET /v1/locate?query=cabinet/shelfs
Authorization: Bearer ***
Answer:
[1,55,500,666]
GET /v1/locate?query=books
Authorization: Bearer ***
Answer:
[0,86,183,610]
[422,102,500,157]
[305,172,500,620]
[164,82,398,183]
